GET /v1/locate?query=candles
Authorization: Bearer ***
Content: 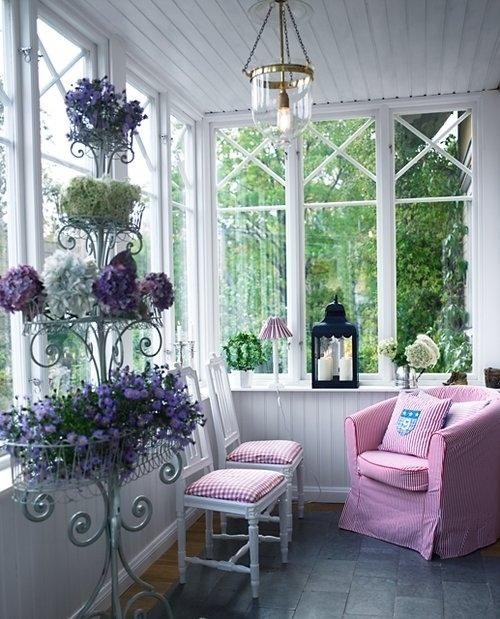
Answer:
[176,320,183,342]
[318,348,354,382]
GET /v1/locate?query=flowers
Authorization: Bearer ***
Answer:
[137,271,176,313]
[0,263,45,320]
[59,174,141,223]
[220,330,268,372]
[63,73,149,145]
[0,361,209,485]
[376,331,441,370]
[39,248,100,319]
[91,259,148,320]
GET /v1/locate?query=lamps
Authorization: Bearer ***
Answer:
[242,1,317,145]
[256,315,295,390]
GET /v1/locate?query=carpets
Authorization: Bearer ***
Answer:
[145,510,500,619]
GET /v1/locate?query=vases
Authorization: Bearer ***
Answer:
[394,366,418,390]
[229,369,254,388]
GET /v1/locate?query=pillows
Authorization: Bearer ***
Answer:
[377,389,454,459]
[414,388,493,430]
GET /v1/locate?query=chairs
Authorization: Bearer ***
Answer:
[337,385,500,561]
[204,351,305,542]
[162,362,289,599]
